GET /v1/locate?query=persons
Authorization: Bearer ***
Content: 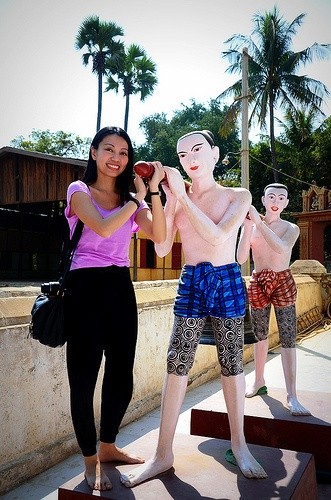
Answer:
[236,183,311,416]
[119,131,269,488]
[64,127,166,490]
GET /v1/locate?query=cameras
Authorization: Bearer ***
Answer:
[41,277,66,296]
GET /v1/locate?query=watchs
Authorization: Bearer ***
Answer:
[126,196,141,209]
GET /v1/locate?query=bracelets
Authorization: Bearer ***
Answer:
[149,191,161,197]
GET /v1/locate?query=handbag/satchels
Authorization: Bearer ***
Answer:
[27,291,68,349]
